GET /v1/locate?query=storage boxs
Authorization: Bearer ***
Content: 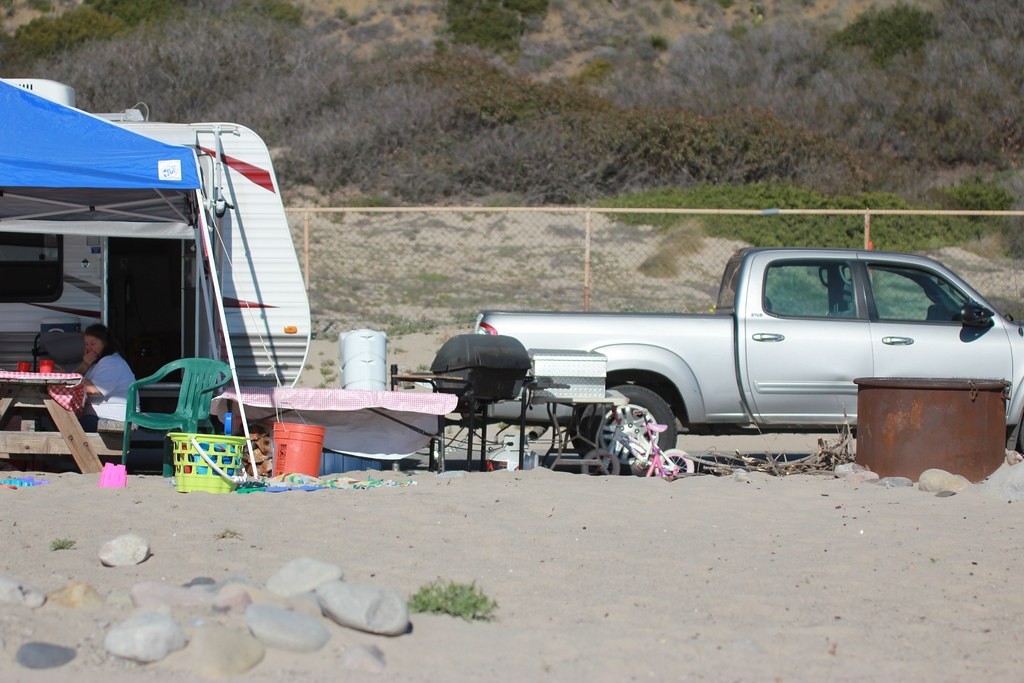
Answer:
[527,349,607,399]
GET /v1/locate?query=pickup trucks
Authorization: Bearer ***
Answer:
[471,245,1023,477]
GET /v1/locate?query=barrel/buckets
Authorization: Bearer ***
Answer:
[271,401,324,480]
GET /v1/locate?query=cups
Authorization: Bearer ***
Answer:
[38,359,54,373]
[17,361,30,372]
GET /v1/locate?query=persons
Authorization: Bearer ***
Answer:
[73,323,141,433]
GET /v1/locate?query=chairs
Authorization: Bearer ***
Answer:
[122,358,241,477]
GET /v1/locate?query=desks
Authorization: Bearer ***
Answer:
[531,388,629,474]
[391,374,537,475]
[0,371,103,473]
[209,386,458,476]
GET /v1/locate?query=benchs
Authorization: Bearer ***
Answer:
[86,419,137,456]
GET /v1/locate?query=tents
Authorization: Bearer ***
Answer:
[0,77,261,482]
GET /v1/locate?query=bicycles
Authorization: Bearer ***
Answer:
[580,404,695,479]
